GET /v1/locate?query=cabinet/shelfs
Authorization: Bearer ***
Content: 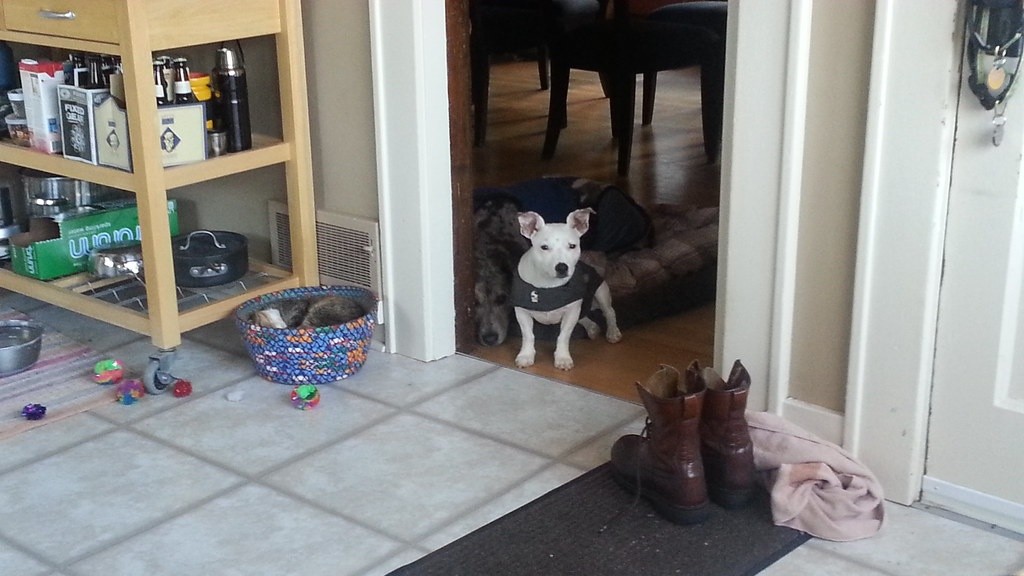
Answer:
[0,0,320,348]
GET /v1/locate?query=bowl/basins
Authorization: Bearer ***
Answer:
[0,319,44,378]
[86,239,145,283]
[4,88,31,147]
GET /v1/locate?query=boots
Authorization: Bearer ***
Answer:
[688,358,758,512]
[610,363,710,525]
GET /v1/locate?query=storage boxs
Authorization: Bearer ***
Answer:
[56,85,109,166]
[19,58,65,155]
[92,96,207,174]
[8,197,180,281]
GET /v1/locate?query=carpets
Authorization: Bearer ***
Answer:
[0,302,141,444]
[386,461,811,576]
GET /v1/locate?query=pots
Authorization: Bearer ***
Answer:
[170,228,250,288]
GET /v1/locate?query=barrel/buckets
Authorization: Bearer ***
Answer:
[18,166,112,219]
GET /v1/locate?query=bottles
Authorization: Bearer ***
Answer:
[61,46,254,156]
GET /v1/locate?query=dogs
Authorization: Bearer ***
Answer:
[248,296,366,329]
[513,207,622,370]
[472,193,533,347]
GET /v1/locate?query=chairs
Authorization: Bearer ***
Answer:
[467,0,727,179]
